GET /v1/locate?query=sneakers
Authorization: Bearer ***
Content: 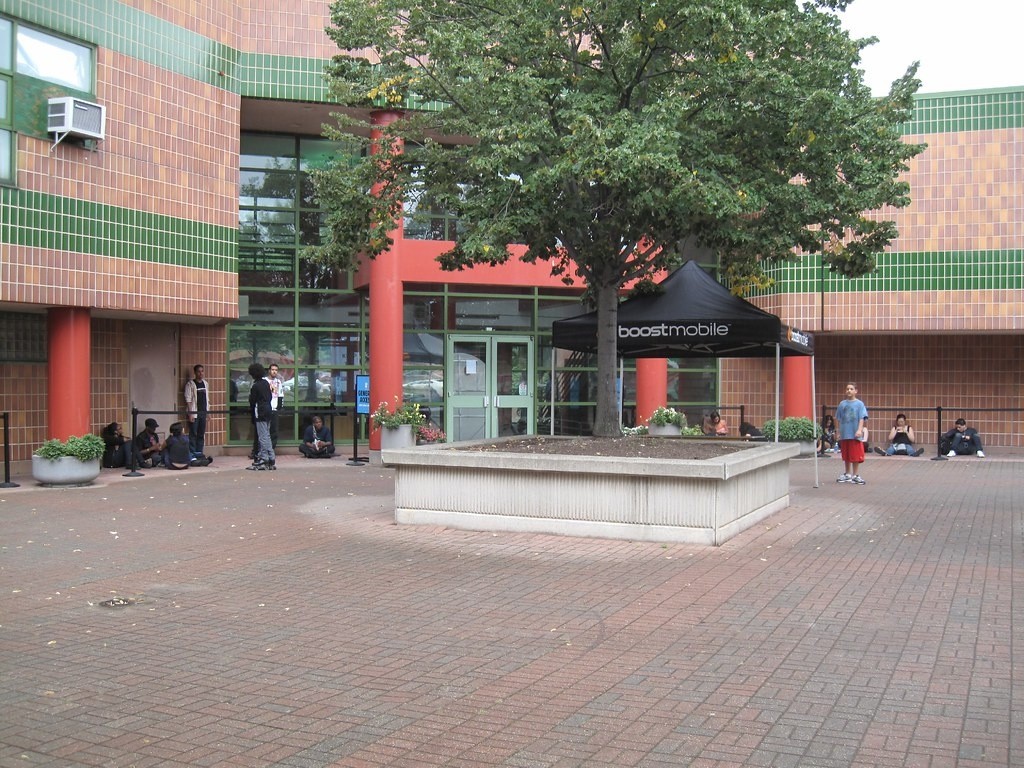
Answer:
[851,475,865,484]
[976,451,985,458]
[947,450,956,457]
[836,473,852,482]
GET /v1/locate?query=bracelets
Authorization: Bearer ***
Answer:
[147,448,150,453]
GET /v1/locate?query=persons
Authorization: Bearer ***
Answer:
[160,422,192,470]
[862,427,869,452]
[706,411,728,436]
[300,415,335,458]
[250,363,284,460]
[817,414,836,453]
[874,414,924,456]
[99,421,141,470]
[184,365,210,456]
[835,382,868,485]
[248,363,275,466]
[738,422,764,441]
[136,418,164,467]
[940,418,985,458]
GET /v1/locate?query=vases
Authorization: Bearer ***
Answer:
[381,423,414,467]
[417,439,442,445]
[648,422,681,435]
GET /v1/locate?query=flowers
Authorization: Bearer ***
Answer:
[621,405,706,438]
[367,393,447,443]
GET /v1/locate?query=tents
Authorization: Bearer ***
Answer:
[551,259,819,487]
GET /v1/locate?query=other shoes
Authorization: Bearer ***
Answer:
[139,462,151,468]
[320,454,333,458]
[246,462,265,470]
[874,446,887,455]
[248,453,256,459]
[127,465,141,470]
[913,448,924,457]
[266,464,276,470]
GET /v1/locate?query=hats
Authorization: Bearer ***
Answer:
[145,418,159,428]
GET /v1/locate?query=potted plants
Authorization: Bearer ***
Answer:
[32,434,106,489]
[765,416,824,458]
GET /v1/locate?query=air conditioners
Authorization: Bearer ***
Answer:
[49,97,107,153]
